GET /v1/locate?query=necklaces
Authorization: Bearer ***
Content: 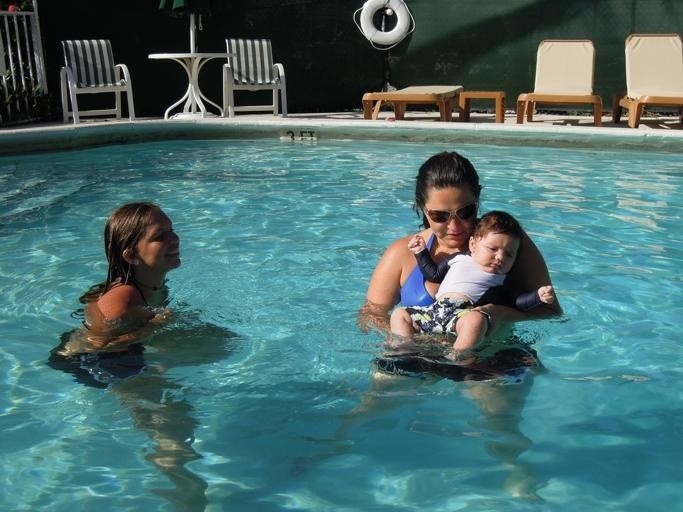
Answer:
[135,280,165,307]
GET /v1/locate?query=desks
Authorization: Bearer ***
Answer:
[148,51,234,121]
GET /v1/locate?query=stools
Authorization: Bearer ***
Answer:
[457,90,506,124]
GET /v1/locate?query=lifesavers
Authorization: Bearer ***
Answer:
[360,0,410,45]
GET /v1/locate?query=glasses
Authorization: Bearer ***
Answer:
[425,203,476,223]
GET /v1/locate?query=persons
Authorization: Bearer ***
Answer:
[46,201,239,512]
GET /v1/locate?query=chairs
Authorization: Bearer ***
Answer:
[221,37,289,119]
[359,83,462,122]
[515,32,683,129]
[58,38,137,124]
